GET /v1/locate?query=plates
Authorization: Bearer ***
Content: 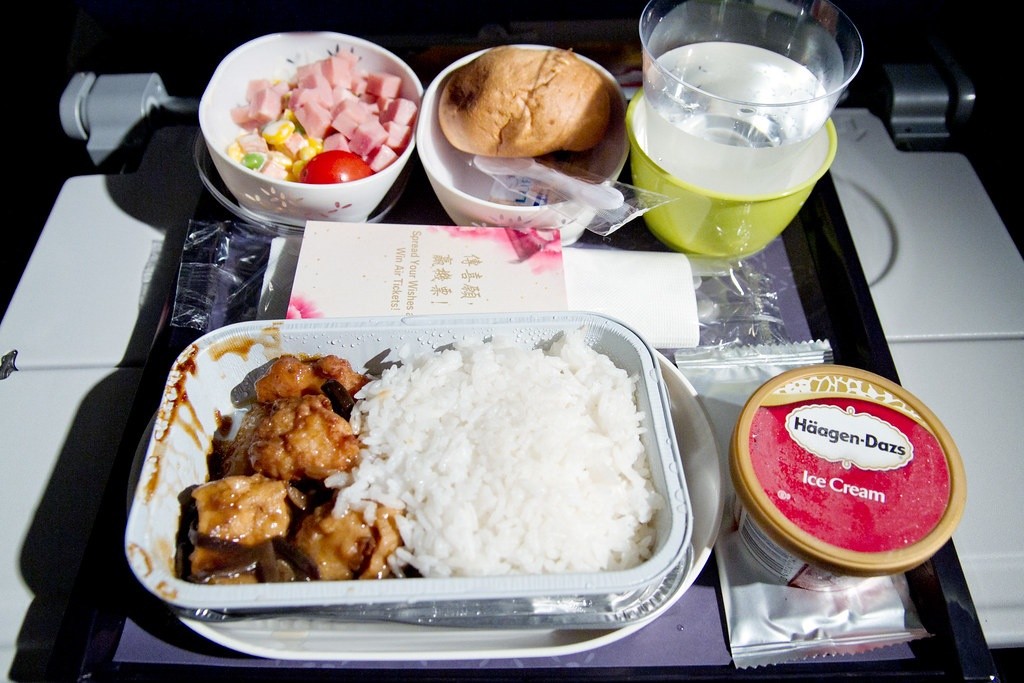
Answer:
[126,341,726,659]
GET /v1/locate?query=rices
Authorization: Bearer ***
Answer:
[324,329,665,578]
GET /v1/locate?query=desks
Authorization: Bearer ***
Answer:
[0,108,1024,683]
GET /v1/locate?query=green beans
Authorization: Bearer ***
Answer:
[293,127,305,136]
[241,153,264,169]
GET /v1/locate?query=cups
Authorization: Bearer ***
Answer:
[199,30,423,221]
[625,88,839,259]
[417,45,631,248]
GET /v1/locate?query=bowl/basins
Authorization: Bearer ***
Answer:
[122,310,694,613]
[639,0,864,150]
[729,365,969,591]
[191,129,413,239]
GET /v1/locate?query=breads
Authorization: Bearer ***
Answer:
[440,47,610,158]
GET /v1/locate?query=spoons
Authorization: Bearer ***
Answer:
[473,154,625,210]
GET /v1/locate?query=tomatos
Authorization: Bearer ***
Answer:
[299,150,375,184]
